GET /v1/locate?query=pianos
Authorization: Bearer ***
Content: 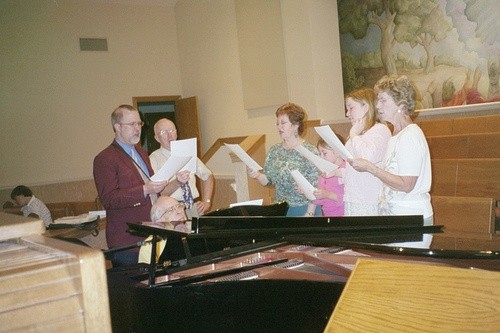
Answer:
[130,202,500,333]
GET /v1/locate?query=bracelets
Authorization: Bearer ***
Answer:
[206,200,211,202]
[375,166,378,176]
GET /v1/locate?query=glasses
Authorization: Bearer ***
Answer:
[159,204,183,220]
[156,128,176,137]
[121,121,144,126]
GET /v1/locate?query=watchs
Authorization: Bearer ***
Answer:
[306,211,314,215]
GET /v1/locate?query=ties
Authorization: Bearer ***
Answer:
[180,182,193,209]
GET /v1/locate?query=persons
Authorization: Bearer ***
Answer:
[320,88,391,216]
[0,185,51,227]
[148,119,213,217]
[248,103,323,216]
[139,196,187,265]
[295,134,345,216]
[347,76,434,249]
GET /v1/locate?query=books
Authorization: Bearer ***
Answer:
[54,214,98,225]
[89,211,106,215]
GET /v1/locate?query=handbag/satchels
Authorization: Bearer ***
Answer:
[49,215,100,231]
